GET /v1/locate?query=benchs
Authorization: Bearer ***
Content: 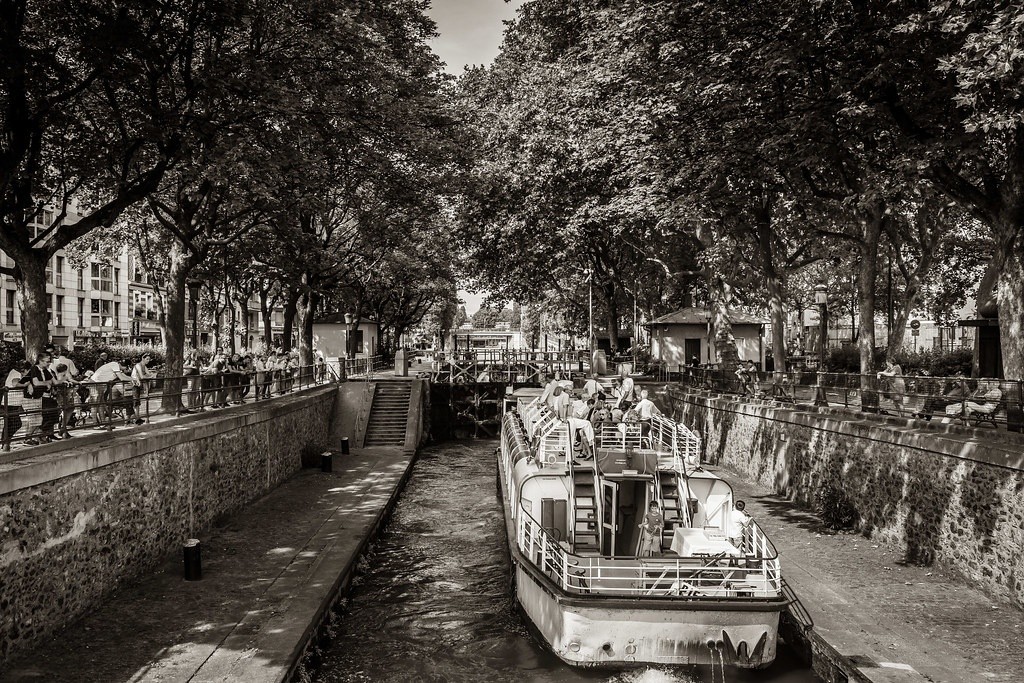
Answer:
[924,393,1008,428]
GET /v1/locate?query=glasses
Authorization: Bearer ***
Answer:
[41,360,50,363]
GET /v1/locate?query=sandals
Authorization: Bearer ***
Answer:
[576,452,586,458]
[584,454,592,461]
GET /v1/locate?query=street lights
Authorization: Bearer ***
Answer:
[814,276,828,407]
[701,301,716,387]
[343,312,352,374]
[187,280,201,406]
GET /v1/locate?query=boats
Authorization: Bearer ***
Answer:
[495,377,782,675]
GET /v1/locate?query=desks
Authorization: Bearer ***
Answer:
[672,526,740,557]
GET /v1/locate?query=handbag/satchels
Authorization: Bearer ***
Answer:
[183,365,193,376]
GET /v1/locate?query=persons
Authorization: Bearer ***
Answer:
[49,349,157,439]
[940,377,1002,427]
[247,351,299,400]
[690,353,699,387]
[572,372,663,461]
[743,359,761,397]
[730,500,754,551]
[609,344,616,361]
[539,371,595,460]
[0,353,71,447]
[875,355,907,417]
[910,371,971,421]
[735,363,750,395]
[316,357,326,383]
[641,501,665,558]
[561,344,586,361]
[183,347,250,412]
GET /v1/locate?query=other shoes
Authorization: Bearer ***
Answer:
[574,446,582,451]
[94,393,275,432]
[3,445,11,451]
[23,439,33,445]
[48,434,62,440]
[574,442,580,447]
[31,438,39,445]
[559,451,565,456]
